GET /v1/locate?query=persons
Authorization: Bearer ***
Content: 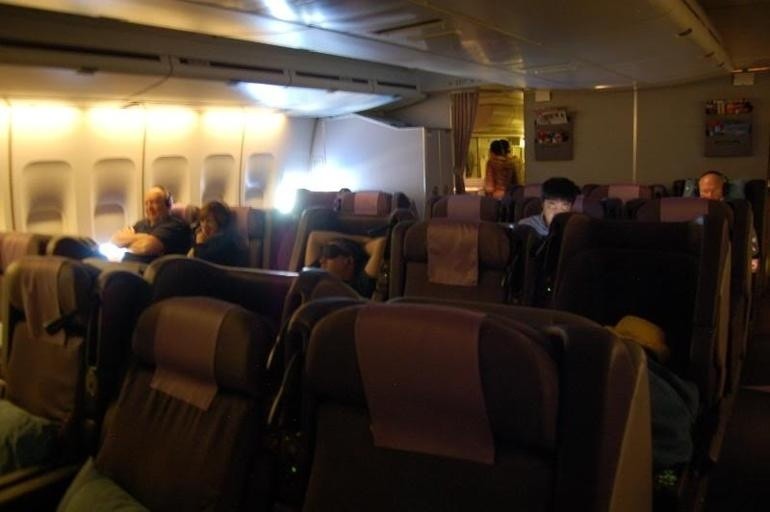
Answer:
[307,239,370,299]
[111,191,193,265]
[389,192,419,222]
[500,140,524,185]
[186,201,250,267]
[518,177,577,238]
[484,140,512,200]
[697,170,760,273]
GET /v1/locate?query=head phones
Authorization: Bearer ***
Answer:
[694,170,729,196]
[154,184,173,207]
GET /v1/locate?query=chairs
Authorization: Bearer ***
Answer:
[583,183,665,208]
[171,205,199,222]
[736,199,753,356]
[1,231,81,258]
[1,258,104,512]
[633,197,736,238]
[388,296,654,512]
[513,183,543,203]
[388,219,539,306]
[232,206,271,267]
[540,212,734,511]
[288,207,417,301]
[515,196,610,221]
[56,254,337,511]
[673,178,767,305]
[336,189,409,216]
[425,194,510,221]
[267,296,568,512]
